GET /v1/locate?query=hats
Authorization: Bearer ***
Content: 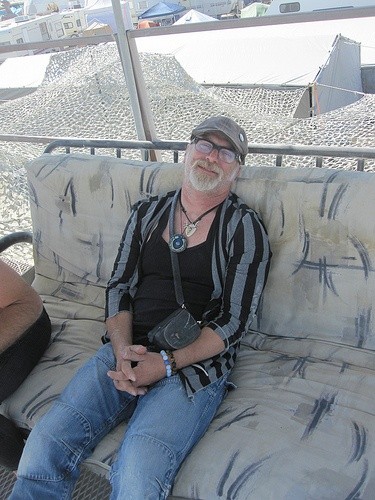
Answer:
[193,118,248,165]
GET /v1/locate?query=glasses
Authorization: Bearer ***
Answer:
[193,135,242,164]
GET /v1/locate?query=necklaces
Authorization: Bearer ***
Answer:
[168,193,191,253]
[178,192,227,238]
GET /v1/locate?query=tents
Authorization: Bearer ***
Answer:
[137,1,186,30]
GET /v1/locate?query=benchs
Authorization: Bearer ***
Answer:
[0,135,375,500]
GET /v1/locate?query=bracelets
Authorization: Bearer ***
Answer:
[160,349,171,378]
[166,350,178,376]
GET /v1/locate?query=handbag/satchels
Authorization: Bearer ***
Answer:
[148,308,201,351]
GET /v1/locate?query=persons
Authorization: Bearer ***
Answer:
[0,256,53,470]
[8,115,274,500]
